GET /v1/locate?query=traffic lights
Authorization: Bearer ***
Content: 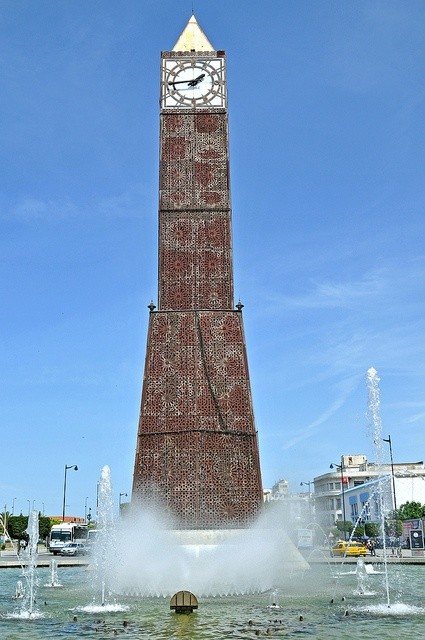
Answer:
[343,477,349,484]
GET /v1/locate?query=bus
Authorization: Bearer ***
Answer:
[50,523,89,555]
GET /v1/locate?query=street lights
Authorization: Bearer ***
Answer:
[42,503,44,516]
[12,498,16,516]
[85,497,88,523]
[62,465,78,522]
[27,500,30,516]
[33,500,35,511]
[119,493,127,521]
[330,462,347,541]
[300,481,311,530]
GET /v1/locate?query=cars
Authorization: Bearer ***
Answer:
[330,541,367,557]
[61,542,85,556]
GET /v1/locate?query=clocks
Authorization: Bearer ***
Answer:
[161,56,227,108]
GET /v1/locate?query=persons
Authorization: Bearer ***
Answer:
[370,540,378,556]
[366,538,372,550]
[65,533,70,540]
